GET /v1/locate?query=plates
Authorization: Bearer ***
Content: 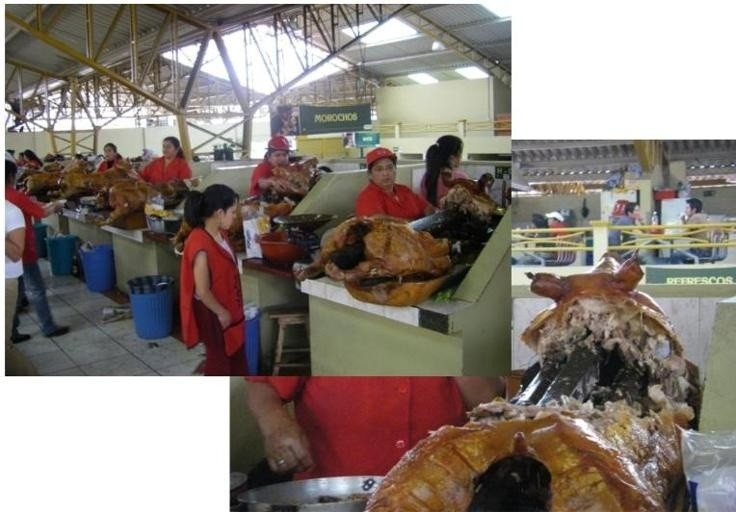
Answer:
[274,213,338,231]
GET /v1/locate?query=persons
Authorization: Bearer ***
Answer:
[14,152,25,166]
[517,213,554,265]
[5,197,37,376]
[136,136,192,184]
[97,143,120,173]
[420,136,469,207]
[344,132,355,148]
[608,202,640,258]
[242,377,508,478]
[5,161,70,343]
[545,212,569,258]
[669,197,707,264]
[355,147,433,223]
[180,184,248,376]
[24,150,42,169]
[250,137,293,195]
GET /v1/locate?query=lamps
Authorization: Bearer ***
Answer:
[431,38,445,51]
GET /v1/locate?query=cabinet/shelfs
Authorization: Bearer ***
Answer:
[300,162,513,377]
[62,206,111,246]
[40,212,68,233]
[174,158,429,364]
[102,157,334,296]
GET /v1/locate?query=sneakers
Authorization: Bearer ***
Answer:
[46,326,69,338]
[11,334,30,344]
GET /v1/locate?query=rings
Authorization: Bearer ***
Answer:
[276,455,284,465]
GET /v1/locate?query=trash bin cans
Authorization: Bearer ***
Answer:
[80,241,117,293]
[44,231,78,275]
[37,224,49,255]
[245,306,263,376]
[127,276,175,341]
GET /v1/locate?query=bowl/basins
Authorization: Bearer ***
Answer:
[258,231,305,265]
[237,476,384,512]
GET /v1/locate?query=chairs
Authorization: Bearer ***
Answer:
[535,232,584,266]
[685,229,729,264]
[266,305,311,376]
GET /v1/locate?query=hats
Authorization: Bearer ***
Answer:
[545,211,565,222]
[367,146,396,165]
[267,135,294,153]
